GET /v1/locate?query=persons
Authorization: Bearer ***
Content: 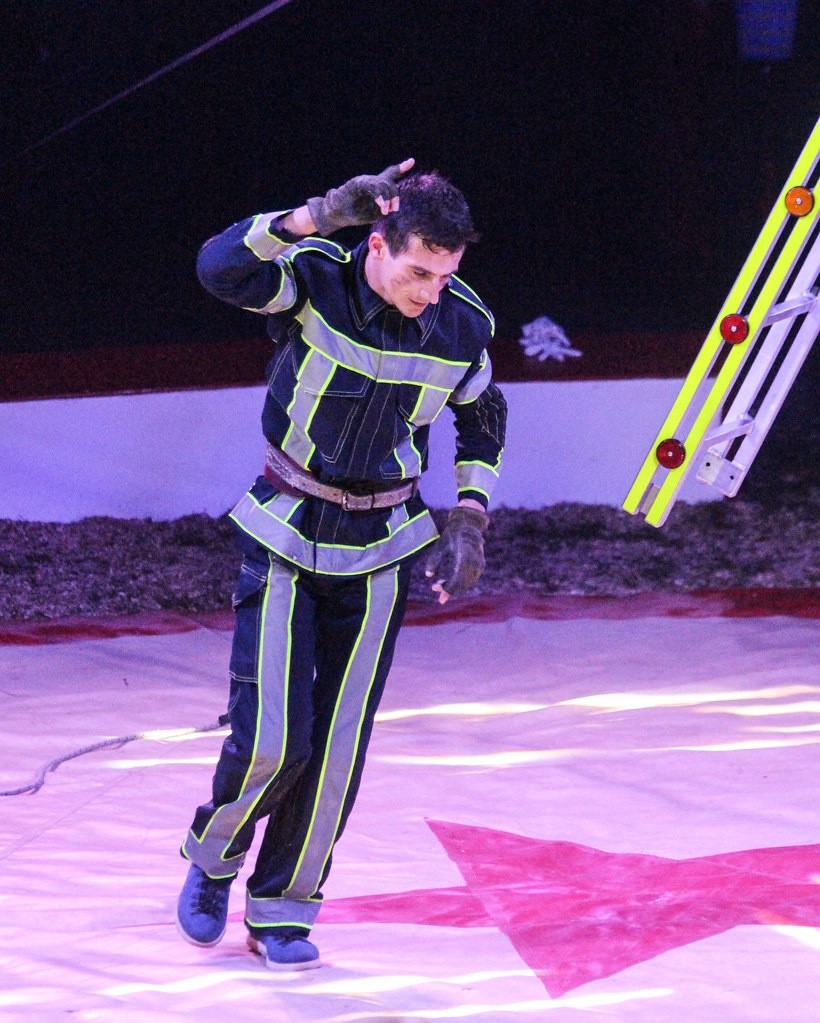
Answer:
[176,158,508,971]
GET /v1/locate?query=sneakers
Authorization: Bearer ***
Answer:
[245,928,322,971]
[175,859,239,948]
